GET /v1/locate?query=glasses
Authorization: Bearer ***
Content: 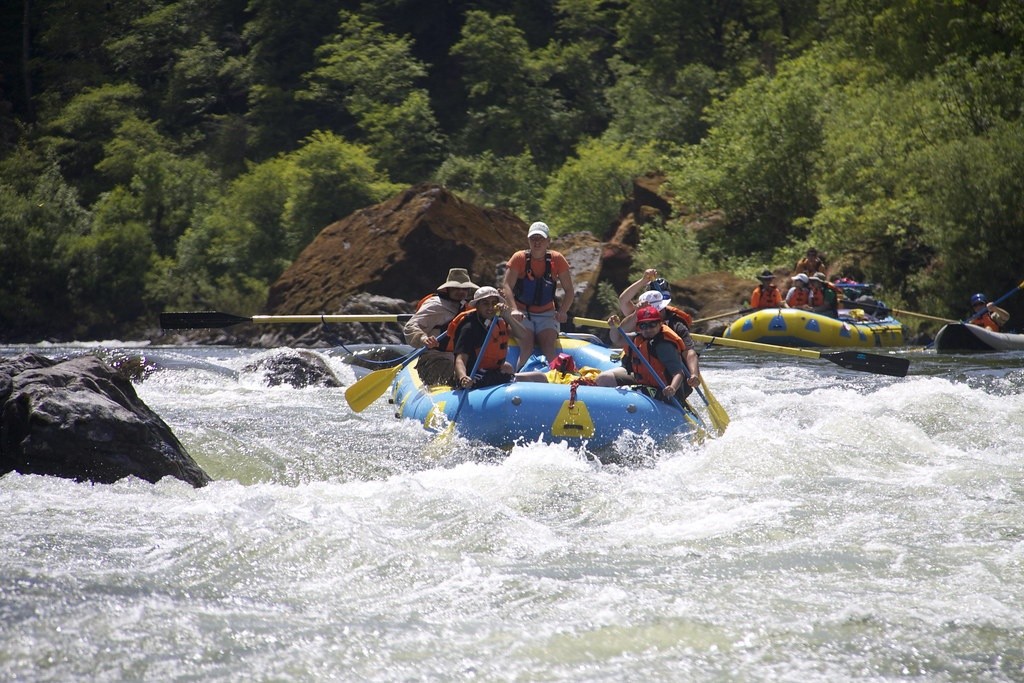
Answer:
[662,294,670,300]
[639,320,660,330]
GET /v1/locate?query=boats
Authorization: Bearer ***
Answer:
[723,307,907,348]
[934,321,1024,352]
[388,336,706,469]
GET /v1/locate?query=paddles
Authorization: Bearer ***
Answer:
[345,331,447,413]
[682,362,727,436]
[828,280,875,288]
[613,316,712,444]
[699,374,730,424]
[159,311,414,332]
[913,281,1024,351]
[692,308,755,324]
[566,315,911,378]
[420,305,502,459]
[838,299,958,323]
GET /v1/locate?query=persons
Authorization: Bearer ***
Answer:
[618,269,692,326]
[502,222,574,370]
[969,294,1010,332]
[403,268,480,386]
[453,286,527,392]
[751,269,782,311]
[802,272,838,319]
[595,307,685,409]
[794,247,825,277]
[607,291,700,387]
[782,273,814,311]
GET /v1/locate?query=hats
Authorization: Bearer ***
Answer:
[756,270,777,279]
[647,278,671,292]
[970,294,987,305]
[527,221,550,239]
[638,290,671,311]
[790,273,809,284]
[468,286,507,307]
[436,268,480,290]
[636,307,664,320]
[809,272,826,284]
